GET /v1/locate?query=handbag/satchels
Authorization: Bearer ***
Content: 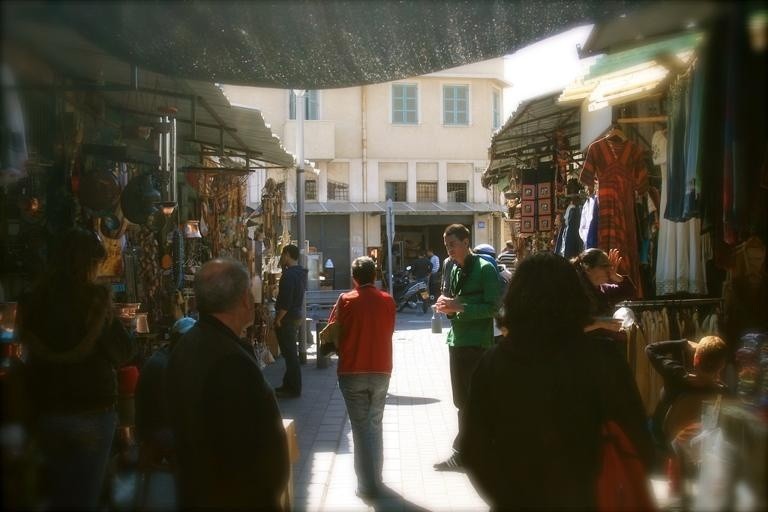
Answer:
[330,320,340,348]
[596,419,659,511]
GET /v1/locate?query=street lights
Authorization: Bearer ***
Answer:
[294,88,308,368]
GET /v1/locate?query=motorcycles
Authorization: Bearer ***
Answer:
[392,265,434,313]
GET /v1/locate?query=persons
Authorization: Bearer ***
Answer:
[431,222,504,470]
[271,245,309,398]
[644,333,732,503]
[383,247,440,315]
[156,256,291,512]
[566,247,638,346]
[455,251,658,511]
[472,244,512,333]
[494,240,517,274]
[7,227,136,512]
[135,315,198,463]
[327,256,398,500]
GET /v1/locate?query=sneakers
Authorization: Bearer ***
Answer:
[355,474,383,498]
[275,386,301,399]
[433,451,465,469]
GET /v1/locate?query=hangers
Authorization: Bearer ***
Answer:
[605,117,628,143]
[623,301,722,322]
